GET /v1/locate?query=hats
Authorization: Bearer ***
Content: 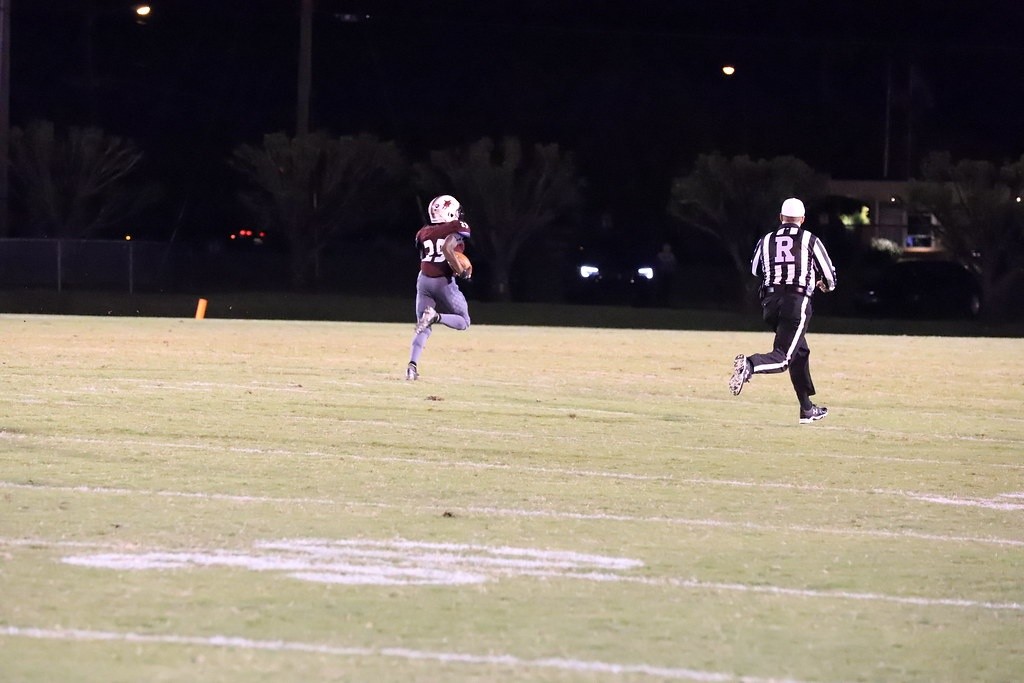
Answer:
[781,198,806,218]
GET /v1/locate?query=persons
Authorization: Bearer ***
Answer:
[729,197,837,424]
[406,195,472,384]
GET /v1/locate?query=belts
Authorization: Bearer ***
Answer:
[764,287,806,294]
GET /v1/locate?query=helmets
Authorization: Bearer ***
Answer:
[428,194,460,223]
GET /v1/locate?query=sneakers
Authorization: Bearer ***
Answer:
[799,401,829,423]
[729,354,752,395]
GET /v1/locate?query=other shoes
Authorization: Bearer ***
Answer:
[415,305,438,335]
[407,361,419,379]
